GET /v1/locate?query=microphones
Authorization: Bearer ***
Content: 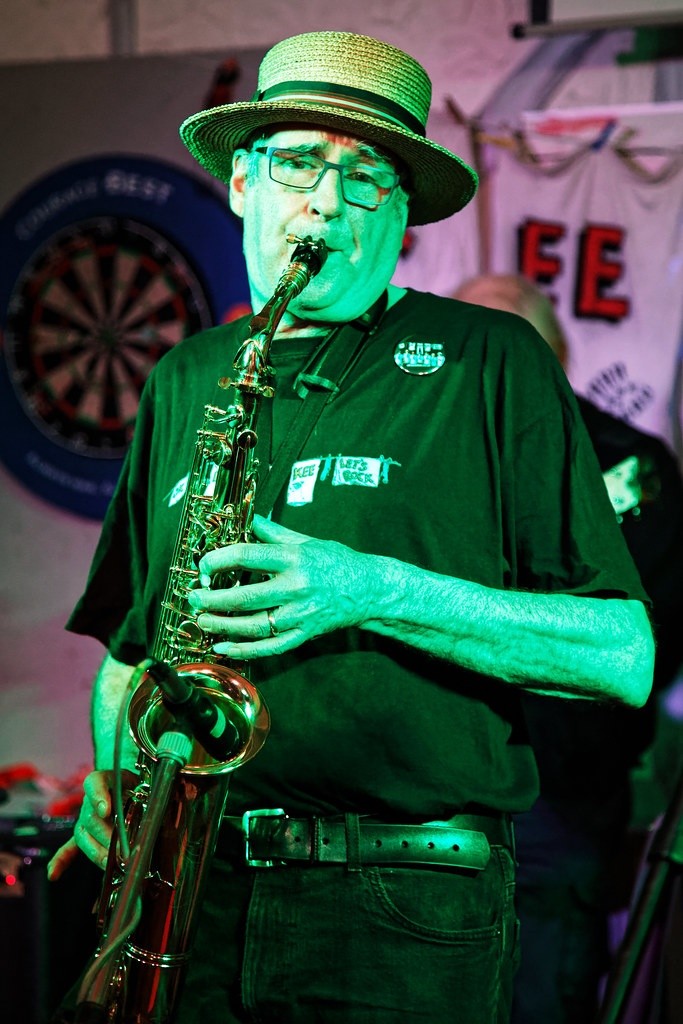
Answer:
[148,659,252,776]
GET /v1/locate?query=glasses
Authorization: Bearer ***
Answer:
[251,147,405,206]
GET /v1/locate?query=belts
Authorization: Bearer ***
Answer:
[221,809,506,871]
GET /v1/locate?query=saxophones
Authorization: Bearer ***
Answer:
[52,237,328,1024]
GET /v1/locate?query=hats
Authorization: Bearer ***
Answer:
[177,31,479,229]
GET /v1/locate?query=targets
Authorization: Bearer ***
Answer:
[15,222,206,459]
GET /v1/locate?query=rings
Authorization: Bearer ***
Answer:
[266,609,279,635]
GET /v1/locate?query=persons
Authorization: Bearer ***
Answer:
[429,270,683,1024]
[46,32,658,1024]
